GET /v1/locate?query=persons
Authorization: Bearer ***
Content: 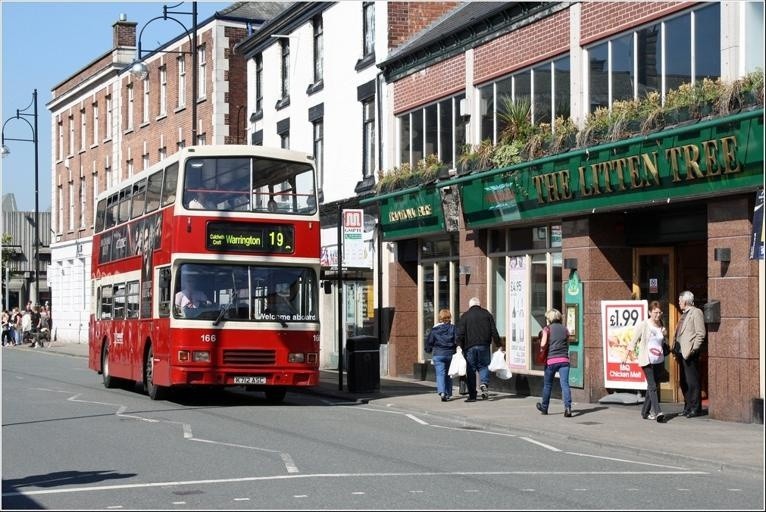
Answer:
[670,291,707,418]
[455,297,501,402]
[174,276,211,318]
[136,214,163,281]
[424,309,455,401]
[268,200,278,212]
[1,301,51,347]
[142,287,153,301]
[189,186,218,210]
[622,301,668,421]
[117,229,126,259]
[536,309,572,417]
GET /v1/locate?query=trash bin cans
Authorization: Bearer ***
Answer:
[346,335,380,394]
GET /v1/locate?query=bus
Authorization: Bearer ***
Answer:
[88,144,331,404]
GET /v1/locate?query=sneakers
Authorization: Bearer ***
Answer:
[441,393,447,401]
[647,412,664,422]
[465,384,488,402]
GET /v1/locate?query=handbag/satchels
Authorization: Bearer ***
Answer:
[449,352,466,377]
[662,343,669,355]
[487,350,512,380]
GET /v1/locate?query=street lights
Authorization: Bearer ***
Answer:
[129,1,198,146]
[0,87,38,302]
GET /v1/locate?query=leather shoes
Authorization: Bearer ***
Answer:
[537,403,547,414]
[677,410,695,417]
[564,408,571,417]
